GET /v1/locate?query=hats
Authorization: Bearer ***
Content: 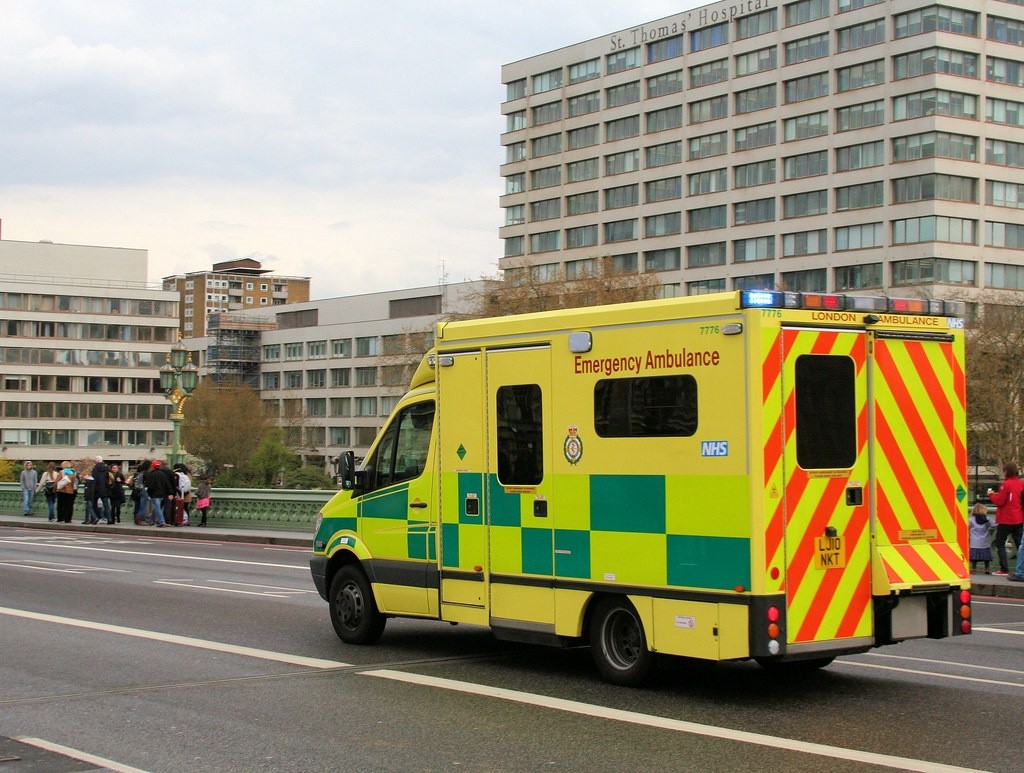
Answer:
[152,461,160,468]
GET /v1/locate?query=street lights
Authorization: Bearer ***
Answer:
[159,332,200,470]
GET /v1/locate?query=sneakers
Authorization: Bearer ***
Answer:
[992,570,1009,576]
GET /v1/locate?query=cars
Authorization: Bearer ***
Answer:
[974,482,1000,504]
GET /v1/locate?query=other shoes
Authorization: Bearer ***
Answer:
[1007,574,1024,582]
[107,522,112,524]
[82,521,90,524]
[984,569,990,574]
[141,521,149,526]
[65,520,72,523]
[50,518,54,521]
[158,524,166,528]
[24,511,27,516]
[117,514,120,523]
[57,519,64,522]
[112,520,116,524]
[970,569,976,574]
[93,518,99,525]
[198,523,206,526]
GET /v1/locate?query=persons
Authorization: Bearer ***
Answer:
[53,461,81,523]
[1007,490,1024,582]
[81,456,126,525]
[194,473,211,527]
[34,462,59,522]
[20,461,37,516]
[968,503,998,574]
[130,459,192,528]
[987,463,1024,576]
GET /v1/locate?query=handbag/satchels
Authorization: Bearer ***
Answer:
[176,507,189,526]
[45,482,56,495]
[196,498,210,509]
[57,471,71,490]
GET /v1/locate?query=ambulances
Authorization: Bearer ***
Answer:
[310,289,970,688]
